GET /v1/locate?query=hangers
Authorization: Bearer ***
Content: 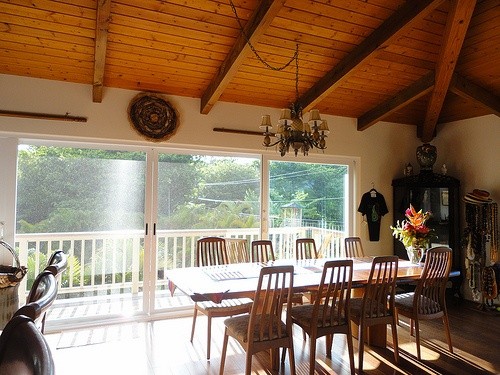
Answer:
[369,182,377,194]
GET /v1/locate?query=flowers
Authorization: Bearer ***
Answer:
[390,204,438,246]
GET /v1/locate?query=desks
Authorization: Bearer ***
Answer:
[163,254,461,347]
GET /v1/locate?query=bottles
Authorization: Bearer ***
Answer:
[406,163,413,176]
[416,143,437,171]
[441,163,447,175]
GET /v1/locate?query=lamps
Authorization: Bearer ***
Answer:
[259,46,330,157]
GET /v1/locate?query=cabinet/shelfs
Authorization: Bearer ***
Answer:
[391,174,460,302]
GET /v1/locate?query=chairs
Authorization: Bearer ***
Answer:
[0,249,68,375]
[191,236,454,375]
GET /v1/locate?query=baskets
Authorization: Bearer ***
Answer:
[0,240,28,331]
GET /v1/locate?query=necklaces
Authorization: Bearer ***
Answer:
[462,202,500,302]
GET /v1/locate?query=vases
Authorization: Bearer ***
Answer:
[407,249,424,263]
